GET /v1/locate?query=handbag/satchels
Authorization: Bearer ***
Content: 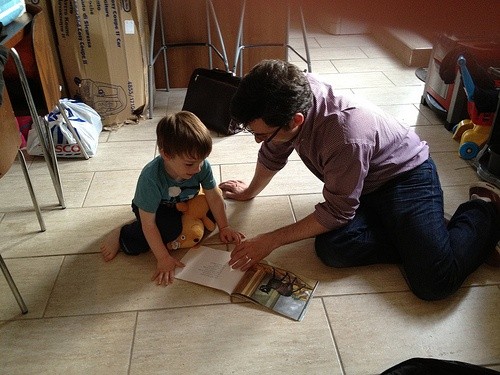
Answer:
[180,67,247,138]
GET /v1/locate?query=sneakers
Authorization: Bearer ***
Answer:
[443,212,500,267]
[467,181,500,207]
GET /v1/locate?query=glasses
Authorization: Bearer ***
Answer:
[243,124,284,145]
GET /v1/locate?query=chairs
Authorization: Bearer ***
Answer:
[0,8,91,315]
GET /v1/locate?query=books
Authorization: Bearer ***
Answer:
[172,243,321,325]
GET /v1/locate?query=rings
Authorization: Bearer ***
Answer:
[244,255,249,260]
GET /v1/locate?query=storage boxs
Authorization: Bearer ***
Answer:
[51,0,154,130]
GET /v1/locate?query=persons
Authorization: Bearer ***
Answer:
[218,60,500,302]
[98,110,246,287]
